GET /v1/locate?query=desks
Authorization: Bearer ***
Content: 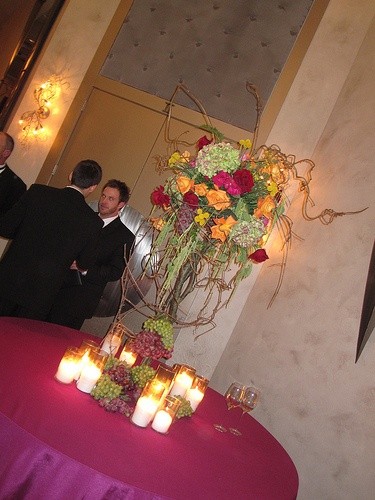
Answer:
[1,311,298,500]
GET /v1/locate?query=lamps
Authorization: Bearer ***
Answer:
[18,66,68,145]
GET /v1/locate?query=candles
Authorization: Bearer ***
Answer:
[55,320,211,435]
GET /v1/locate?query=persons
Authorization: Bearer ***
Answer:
[45,179,134,330]
[0,160,103,321]
[0,132,26,217]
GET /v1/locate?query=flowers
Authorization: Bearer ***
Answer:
[118,82,369,346]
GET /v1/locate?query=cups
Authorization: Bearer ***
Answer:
[131,362,209,434]
[101,328,125,357]
[120,337,138,367]
[73,339,100,383]
[55,345,84,384]
[76,349,109,394]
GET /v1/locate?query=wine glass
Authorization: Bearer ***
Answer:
[213,382,245,433]
[229,387,260,437]
